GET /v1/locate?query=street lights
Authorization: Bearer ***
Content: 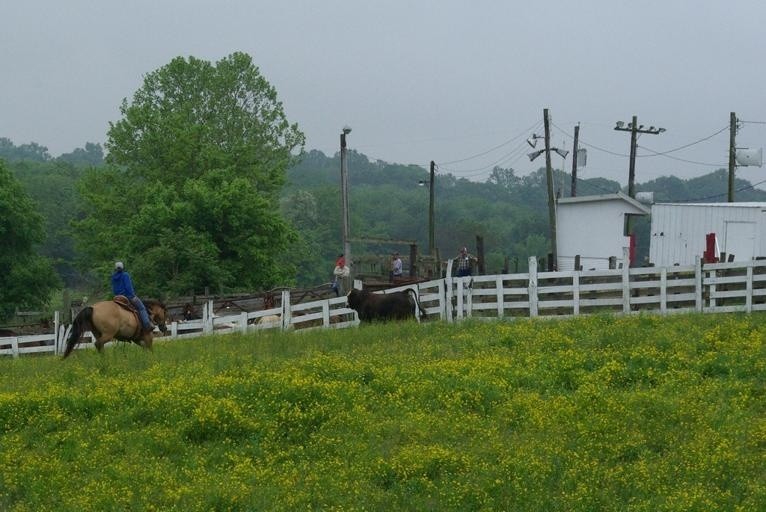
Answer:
[419,161,435,256]
[614,115,665,235]
[527,109,569,273]
[340,126,352,266]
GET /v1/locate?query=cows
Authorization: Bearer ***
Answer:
[344,288,429,322]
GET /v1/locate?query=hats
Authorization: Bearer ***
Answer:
[114,263,124,268]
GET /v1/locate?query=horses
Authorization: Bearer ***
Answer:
[64,299,168,358]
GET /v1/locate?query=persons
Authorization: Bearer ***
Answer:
[336,253,345,266]
[388,253,403,283]
[441,247,478,300]
[332,262,350,296]
[111,261,156,332]
